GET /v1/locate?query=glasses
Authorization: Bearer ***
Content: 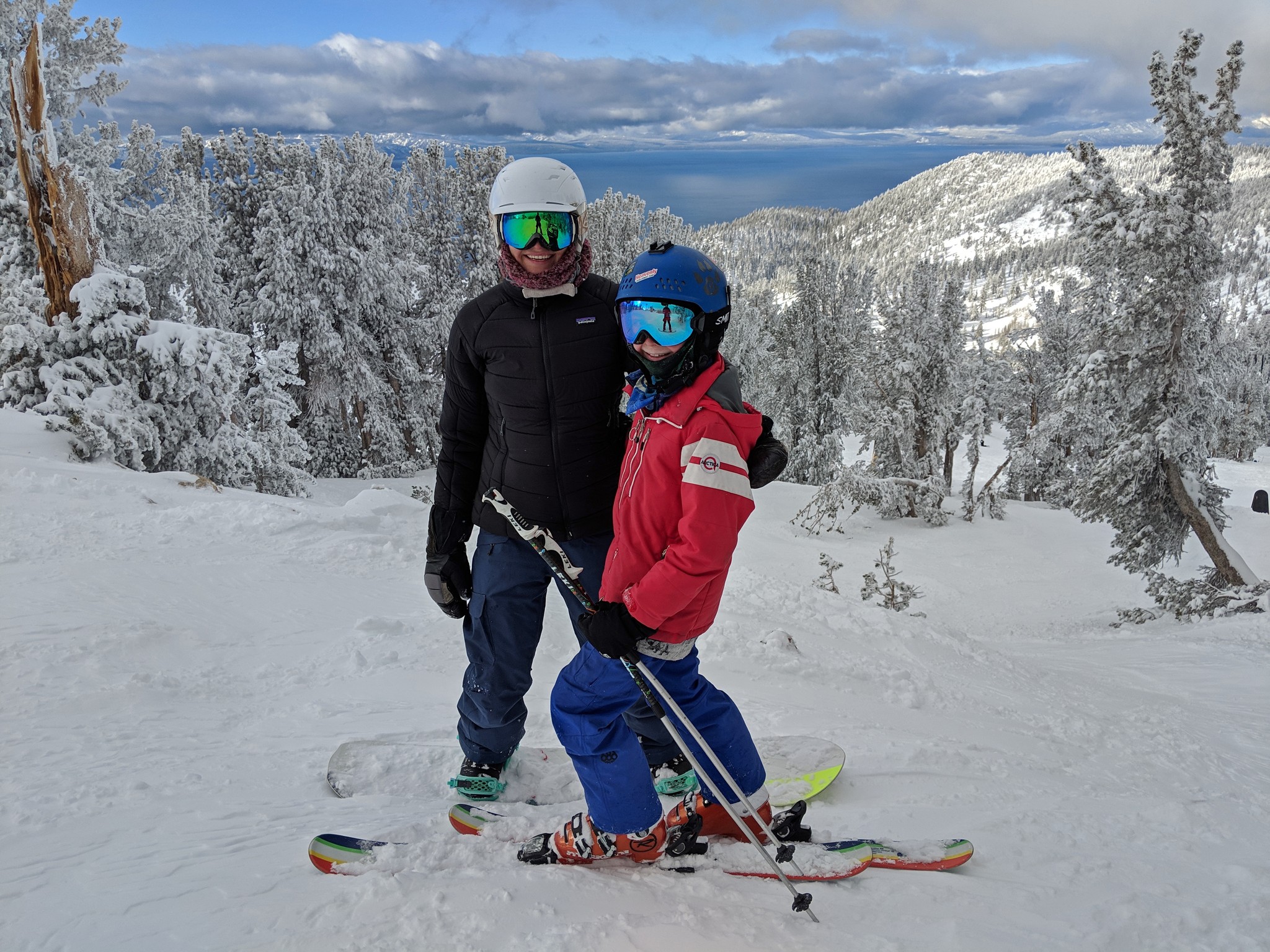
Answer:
[497,211,578,251]
[618,299,697,347]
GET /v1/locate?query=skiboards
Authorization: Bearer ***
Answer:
[307,802,975,883]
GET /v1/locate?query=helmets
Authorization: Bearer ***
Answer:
[614,244,731,346]
[487,157,587,254]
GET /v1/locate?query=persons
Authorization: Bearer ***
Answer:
[535,212,543,235]
[548,239,763,864]
[422,158,791,800]
[662,304,672,332]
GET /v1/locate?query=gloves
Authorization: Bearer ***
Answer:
[577,601,659,659]
[746,415,788,489]
[424,504,472,619]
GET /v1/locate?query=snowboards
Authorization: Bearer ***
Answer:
[328,735,845,808]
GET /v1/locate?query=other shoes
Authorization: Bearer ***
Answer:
[458,751,506,800]
[650,751,693,793]
[667,793,772,845]
[550,815,670,864]
